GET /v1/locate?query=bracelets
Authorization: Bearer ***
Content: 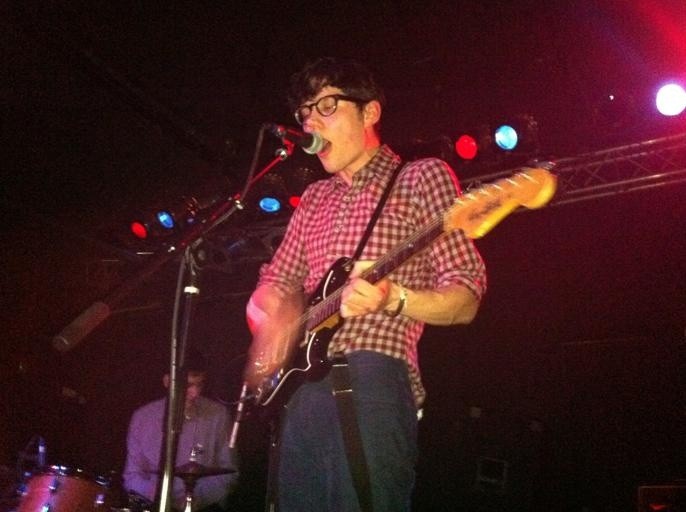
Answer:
[384,289,409,319]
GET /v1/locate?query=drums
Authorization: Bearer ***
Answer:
[14,465,129,512]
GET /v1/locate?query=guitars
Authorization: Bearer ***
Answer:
[245,160,557,407]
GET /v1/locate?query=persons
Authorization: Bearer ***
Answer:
[120,343,241,511]
[241,51,491,511]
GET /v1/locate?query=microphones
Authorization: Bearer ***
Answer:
[262,121,324,154]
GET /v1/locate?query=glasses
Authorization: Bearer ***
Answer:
[294,95,365,125]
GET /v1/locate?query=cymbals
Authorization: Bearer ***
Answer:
[144,464,236,477]
